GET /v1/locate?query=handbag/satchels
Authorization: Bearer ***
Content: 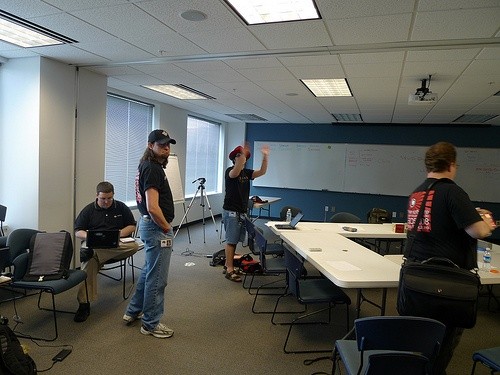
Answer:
[396,257,478,330]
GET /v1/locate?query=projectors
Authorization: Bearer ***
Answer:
[407,93,438,105]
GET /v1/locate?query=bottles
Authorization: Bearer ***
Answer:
[483,247,490,272]
[287,209,291,222]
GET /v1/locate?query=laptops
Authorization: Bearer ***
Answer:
[275,213,304,229]
[86,230,120,249]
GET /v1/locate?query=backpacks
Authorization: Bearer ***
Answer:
[0,324,37,375]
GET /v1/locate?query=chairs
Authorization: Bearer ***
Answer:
[367,208,405,256]
[0,229,90,342]
[332,316,446,375]
[80,220,139,300]
[471,346,500,375]
[274,207,302,245]
[249,229,307,314]
[271,243,352,353]
[242,220,288,289]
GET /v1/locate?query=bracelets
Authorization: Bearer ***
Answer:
[264,157,267,160]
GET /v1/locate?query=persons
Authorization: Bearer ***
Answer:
[396,142,495,375]
[73,182,139,322]
[123,130,177,338]
[222,144,270,282]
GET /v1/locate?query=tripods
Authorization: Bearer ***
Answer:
[173,178,221,244]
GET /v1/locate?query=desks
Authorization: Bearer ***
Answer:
[266,221,500,345]
[249,196,282,223]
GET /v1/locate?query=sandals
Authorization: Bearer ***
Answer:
[223,266,242,274]
[226,269,242,282]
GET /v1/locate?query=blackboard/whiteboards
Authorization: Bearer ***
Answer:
[161,153,186,204]
[252,140,500,203]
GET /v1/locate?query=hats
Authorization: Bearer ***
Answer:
[149,129,176,144]
[229,146,251,160]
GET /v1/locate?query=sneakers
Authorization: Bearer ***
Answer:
[74,303,90,321]
[123,312,144,321]
[79,248,94,261]
[141,322,174,338]
